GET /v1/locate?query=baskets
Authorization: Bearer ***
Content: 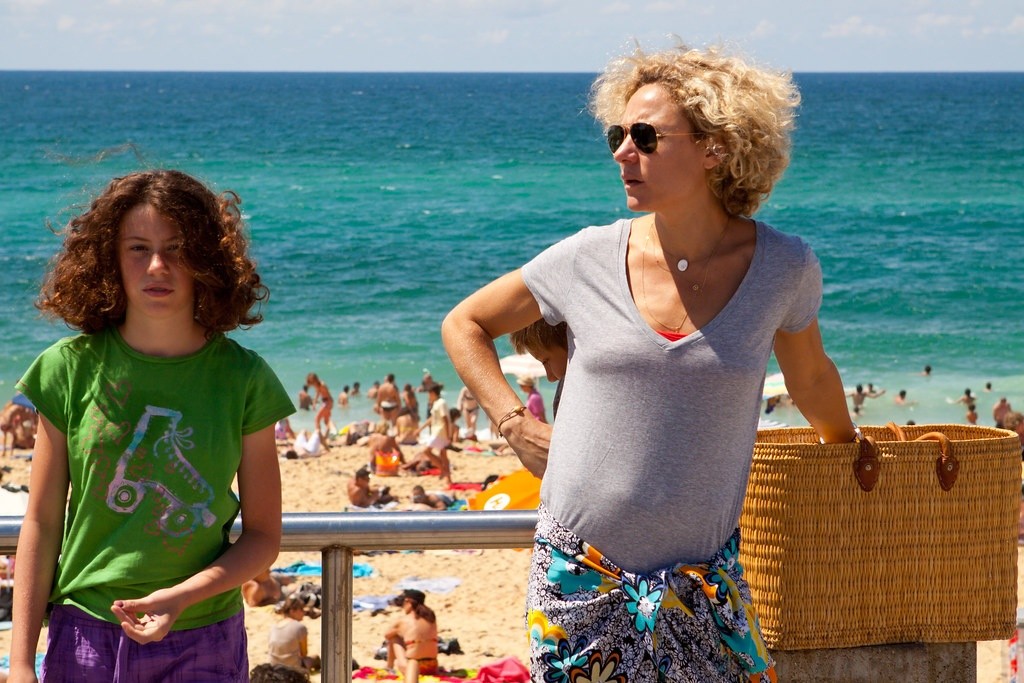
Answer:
[738,423,1022,649]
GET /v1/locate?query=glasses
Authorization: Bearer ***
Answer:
[607,122,709,153]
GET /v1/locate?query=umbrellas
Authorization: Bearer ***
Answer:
[497,351,546,389]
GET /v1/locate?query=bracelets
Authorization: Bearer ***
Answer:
[496,405,527,437]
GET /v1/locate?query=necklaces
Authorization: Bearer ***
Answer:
[642,221,735,332]
[654,213,731,291]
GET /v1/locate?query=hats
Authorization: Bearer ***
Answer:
[395,589,425,604]
[356,470,371,477]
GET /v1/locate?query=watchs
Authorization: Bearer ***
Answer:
[819,422,862,446]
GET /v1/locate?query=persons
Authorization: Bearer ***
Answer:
[437,34,865,683]
[0,169,297,683]
[0,318,1024,683]
